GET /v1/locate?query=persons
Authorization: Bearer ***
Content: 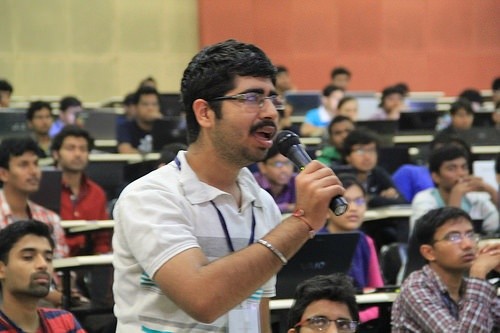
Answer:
[315,171,385,331]
[323,127,407,210]
[482,78,499,113]
[0,218,88,333]
[273,64,295,117]
[286,273,362,333]
[0,136,116,332]
[409,145,499,248]
[428,101,482,148]
[493,162,500,194]
[482,99,500,145]
[301,84,346,136]
[50,125,110,221]
[117,91,181,181]
[390,206,500,333]
[0,64,500,241]
[393,137,471,206]
[138,75,157,91]
[112,39,346,332]
[252,145,299,215]
[315,115,356,168]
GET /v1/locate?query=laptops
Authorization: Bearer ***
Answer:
[355,121,393,143]
[285,93,321,117]
[270,231,361,301]
[0,109,29,135]
[399,111,437,135]
[75,110,117,140]
[28,171,62,216]
[159,94,182,117]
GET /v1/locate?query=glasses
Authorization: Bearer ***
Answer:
[207,91,287,110]
[268,161,294,168]
[293,316,359,333]
[432,231,478,243]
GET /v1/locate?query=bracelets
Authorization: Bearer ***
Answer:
[253,237,289,266]
[291,208,318,239]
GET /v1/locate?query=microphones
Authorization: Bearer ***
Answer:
[276,131,349,216]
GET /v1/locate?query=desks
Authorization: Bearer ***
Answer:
[22,92,500,333]
